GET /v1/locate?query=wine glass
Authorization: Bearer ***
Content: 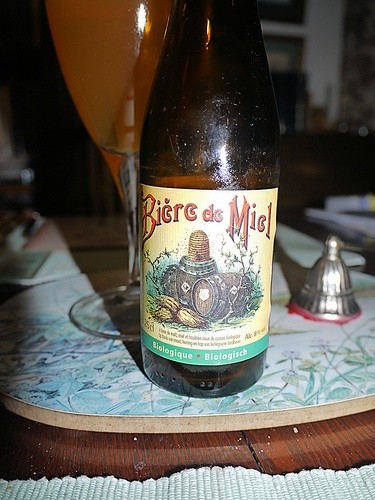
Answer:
[45,0,175,340]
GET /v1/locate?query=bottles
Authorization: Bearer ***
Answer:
[139,0,280,398]
